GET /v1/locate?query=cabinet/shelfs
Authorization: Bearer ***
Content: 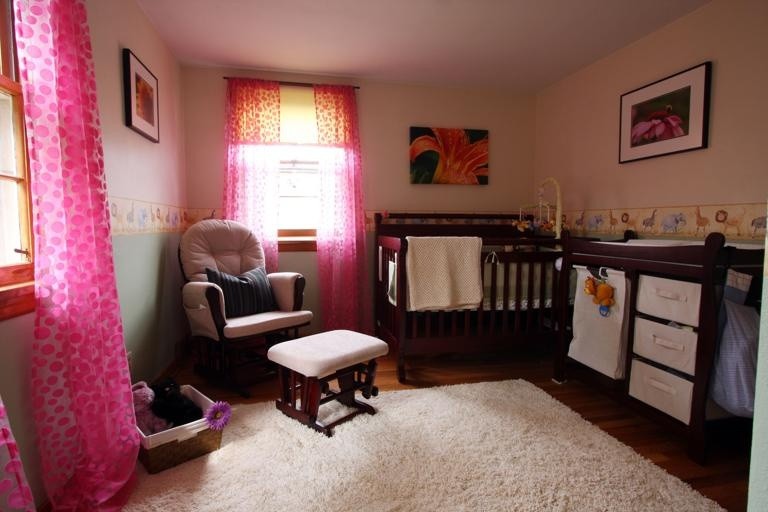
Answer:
[559,221,765,452]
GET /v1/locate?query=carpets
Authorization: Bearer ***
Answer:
[96,377,725,512]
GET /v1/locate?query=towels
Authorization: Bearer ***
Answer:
[390,234,484,312]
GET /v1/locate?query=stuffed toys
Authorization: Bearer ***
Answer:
[131,375,203,437]
[512,214,566,235]
[584,278,615,306]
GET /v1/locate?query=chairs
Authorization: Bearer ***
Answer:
[177,217,312,398]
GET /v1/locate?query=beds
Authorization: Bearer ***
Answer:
[375,208,602,384]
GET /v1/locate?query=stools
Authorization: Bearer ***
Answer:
[266,329,389,438]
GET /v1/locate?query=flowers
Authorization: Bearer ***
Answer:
[206,402,232,432]
[409,127,489,185]
[631,106,684,143]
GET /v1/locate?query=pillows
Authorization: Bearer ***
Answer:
[204,264,281,318]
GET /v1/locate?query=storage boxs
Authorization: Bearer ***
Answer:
[127,382,229,474]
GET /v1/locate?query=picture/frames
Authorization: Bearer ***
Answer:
[122,47,159,144]
[618,60,714,165]
